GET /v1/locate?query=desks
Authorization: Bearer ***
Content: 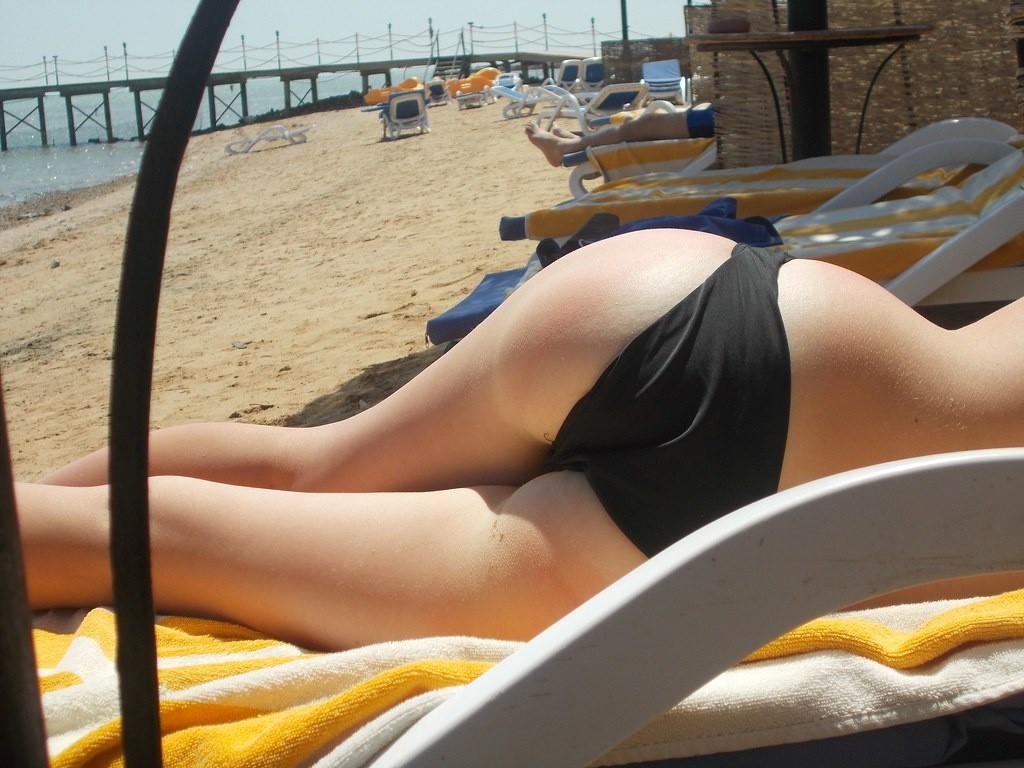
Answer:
[681,23,935,165]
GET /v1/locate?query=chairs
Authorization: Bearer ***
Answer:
[424,139,1024,355]
[378,90,431,141]
[456,84,497,111]
[34,449,1024,768]
[589,100,711,129]
[541,58,583,94]
[579,57,603,90]
[491,85,600,119]
[640,59,686,105]
[493,73,524,91]
[499,117,1024,246]
[224,122,317,155]
[534,82,650,135]
[425,80,456,109]
[562,136,717,197]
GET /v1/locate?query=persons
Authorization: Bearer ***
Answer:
[525,105,715,167]
[16,230,1024,652]
